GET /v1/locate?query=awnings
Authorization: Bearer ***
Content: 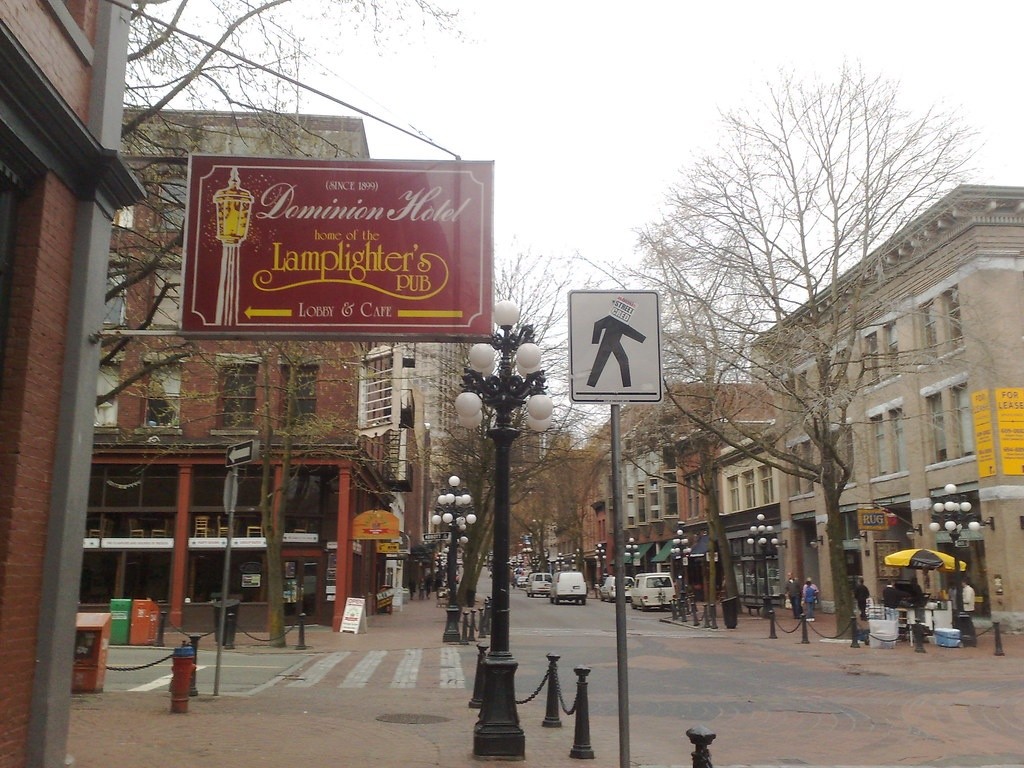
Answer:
[650,541,675,562]
[692,535,718,553]
[610,543,653,566]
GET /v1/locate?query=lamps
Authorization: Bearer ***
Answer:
[906,524,922,539]
[810,536,823,549]
[852,531,867,544]
[775,539,788,550]
[980,516,995,530]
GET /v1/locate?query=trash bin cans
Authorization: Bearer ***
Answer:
[436,587,449,608]
[721,596,737,629]
[214,599,239,643]
[130,600,160,645]
[108,598,131,645]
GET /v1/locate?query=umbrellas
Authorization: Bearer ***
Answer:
[884,548,967,592]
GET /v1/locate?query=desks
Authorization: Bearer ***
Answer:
[920,607,946,641]
[895,608,918,646]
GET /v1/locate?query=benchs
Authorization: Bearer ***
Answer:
[745,604,763,616]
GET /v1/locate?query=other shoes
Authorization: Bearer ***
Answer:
[806,618,815,622]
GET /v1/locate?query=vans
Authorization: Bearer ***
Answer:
[525,573,552,598]
[550,572,587,605]
[670,529,692,601]
[630,572,677,612]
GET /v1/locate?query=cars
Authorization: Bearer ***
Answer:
[513,574,526,588]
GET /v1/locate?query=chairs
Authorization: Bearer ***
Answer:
[91,517,310,539]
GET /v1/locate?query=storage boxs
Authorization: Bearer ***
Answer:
[868,606,899,649]
[928,627,961,647]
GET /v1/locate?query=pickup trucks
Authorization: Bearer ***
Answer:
[599,577,635,603]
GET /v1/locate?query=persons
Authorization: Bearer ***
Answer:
[948,580,977,646]
[409,572,442,600]
[883,580,897,608]
[854,578,869,621]
[784,573,819,621]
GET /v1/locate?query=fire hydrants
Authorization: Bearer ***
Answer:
[167,646,198,714]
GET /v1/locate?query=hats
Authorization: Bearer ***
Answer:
[806,577,812,582]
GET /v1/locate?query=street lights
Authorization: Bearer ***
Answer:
[452,301,552,763]
[746,514,780,617]
[593,544,607,584]
[929,484,980,612]
[625,538,640,576]
[510,549,580,571]
[433,476,477,644]
